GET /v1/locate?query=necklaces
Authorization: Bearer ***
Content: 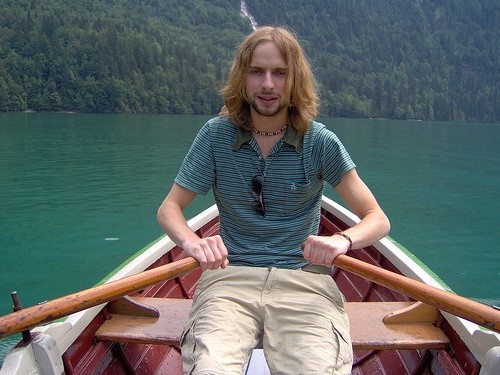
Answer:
[251,122,289,137]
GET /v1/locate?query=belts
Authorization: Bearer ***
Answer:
[302,265,331,274]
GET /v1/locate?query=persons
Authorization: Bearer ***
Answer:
[157,25,391,375]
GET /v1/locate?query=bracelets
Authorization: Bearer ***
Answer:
[333,230,352,251]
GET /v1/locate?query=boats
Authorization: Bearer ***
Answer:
[0,188,500,375]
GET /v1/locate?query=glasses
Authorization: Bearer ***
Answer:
[251,175,266,218]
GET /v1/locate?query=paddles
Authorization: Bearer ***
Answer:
[300,240,500,335]
[0,256,201,338]
